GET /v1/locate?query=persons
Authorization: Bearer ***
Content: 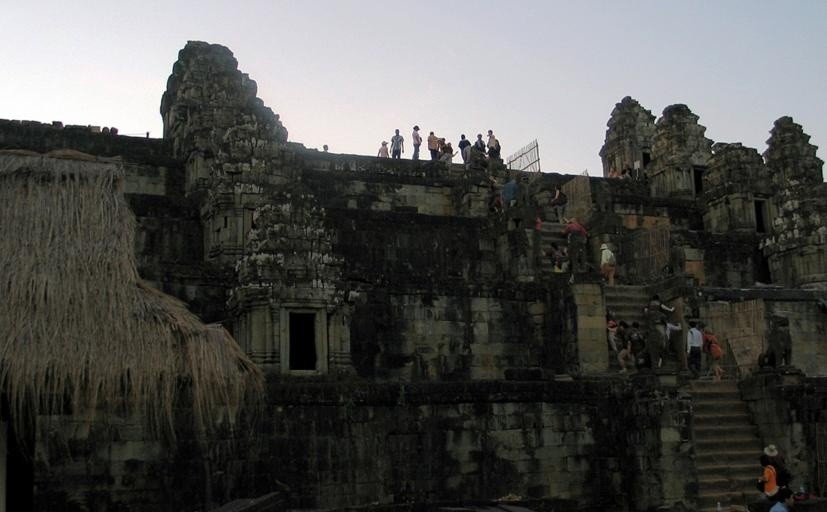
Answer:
[492,175,568,225]
[549,218,616,285]
[608,295,724,381]
[377,126,500,172]
[322,144,328,152]
[757,444,795,512]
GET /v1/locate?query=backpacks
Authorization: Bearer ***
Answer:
[607,250,616,267]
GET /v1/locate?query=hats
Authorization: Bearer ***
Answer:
[763,444,779,458]
[599,243,609,250]
